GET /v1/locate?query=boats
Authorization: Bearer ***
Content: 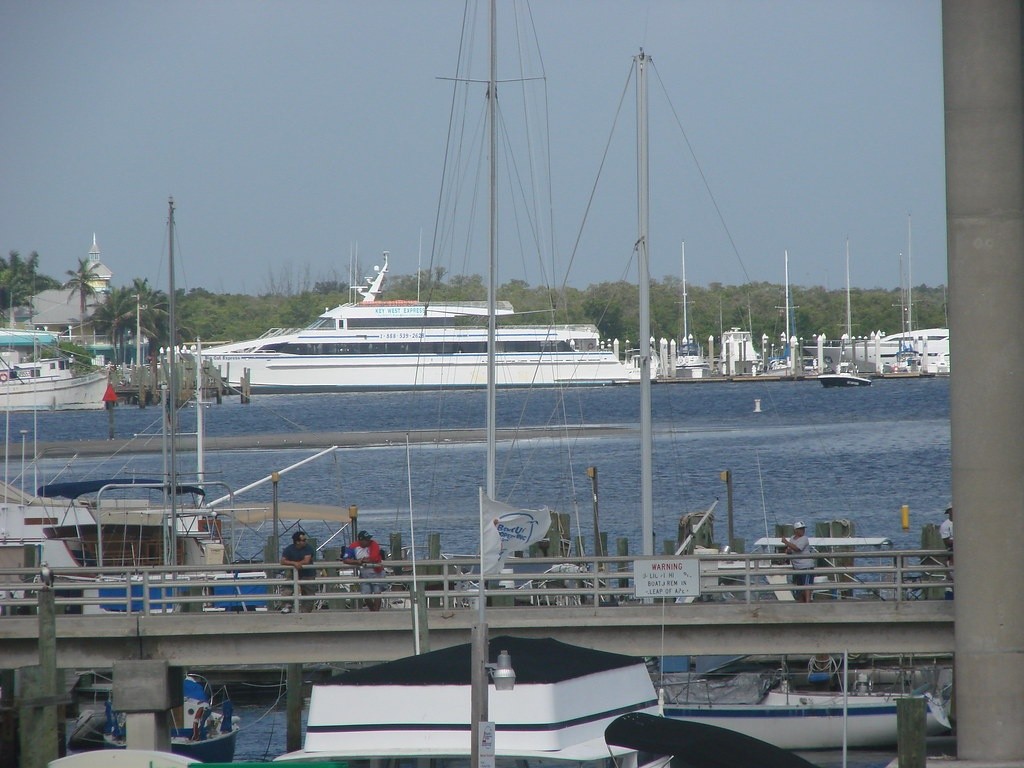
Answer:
[802,328,950,373]
[0,334,952,768]
[0,358,112,412]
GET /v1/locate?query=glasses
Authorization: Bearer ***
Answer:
[300,538,306,542]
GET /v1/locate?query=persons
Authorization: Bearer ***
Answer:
[782,520,815,602]
[939,503,955,600]
[343,530,384,612]
[281,531,316,613]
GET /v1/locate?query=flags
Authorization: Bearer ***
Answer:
[480,490,552,576]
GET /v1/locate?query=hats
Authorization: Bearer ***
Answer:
[944,502,952,514]
[358,530,373,541]
[793,521,807,529]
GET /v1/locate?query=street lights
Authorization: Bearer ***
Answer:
[130,294,140,371]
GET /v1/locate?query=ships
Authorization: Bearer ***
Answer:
[193,226,663,394]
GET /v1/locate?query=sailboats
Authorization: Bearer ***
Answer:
[817,237,872,388]
[759,249,796,378]
[674,240,703,367]
[882,213,924,373]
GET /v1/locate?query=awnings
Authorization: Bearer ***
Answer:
[0,335,57,346]
